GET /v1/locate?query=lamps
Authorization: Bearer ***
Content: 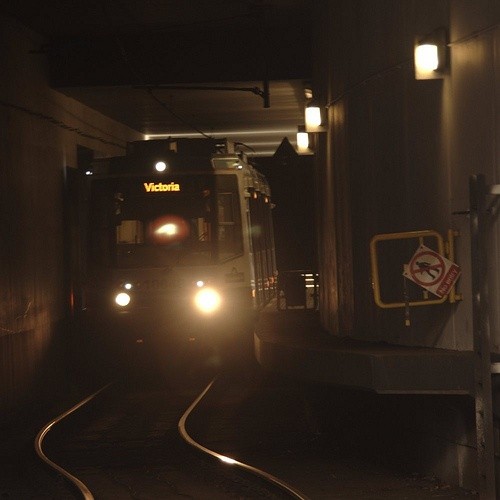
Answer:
[296,124,317,155]
[413,33,447,81]
[304,101,329,133]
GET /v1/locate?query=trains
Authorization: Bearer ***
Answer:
[67,133,273,390]
